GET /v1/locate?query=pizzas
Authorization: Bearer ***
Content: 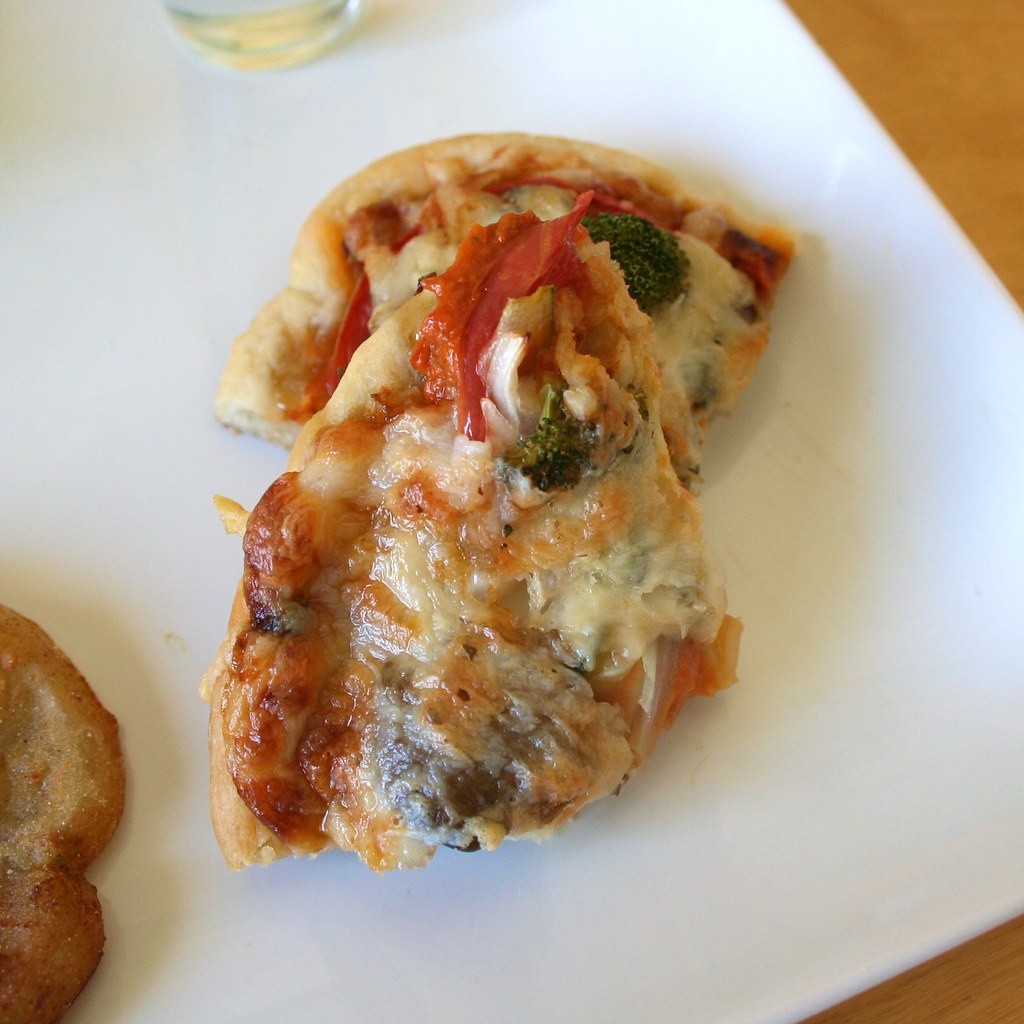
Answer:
[212,134,799,492]
[200,186,747,867]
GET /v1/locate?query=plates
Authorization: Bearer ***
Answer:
[0,1,1024,1023]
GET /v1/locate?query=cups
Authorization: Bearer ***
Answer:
[161,0,360,73]
